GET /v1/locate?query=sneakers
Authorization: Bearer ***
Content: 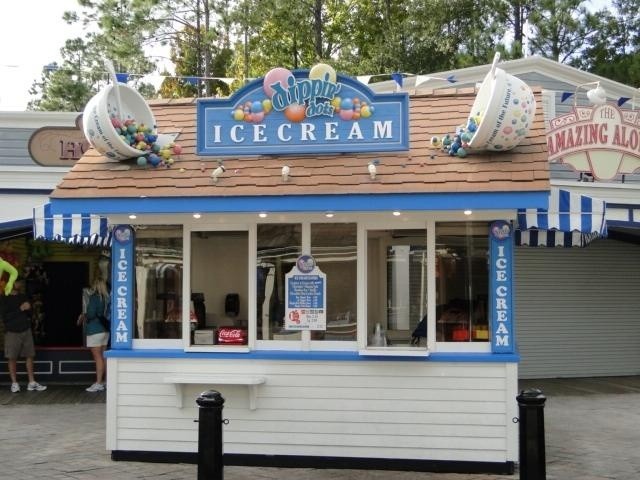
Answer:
[27,382,47,391]
[11,382,20,393]
[85,383,104,392]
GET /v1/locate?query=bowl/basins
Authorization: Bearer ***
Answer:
[458,67,539,154]
[81,83,159,163]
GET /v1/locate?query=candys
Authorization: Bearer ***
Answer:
[430,113,483,159]
[230,94,377,124]
[109,115,182,171]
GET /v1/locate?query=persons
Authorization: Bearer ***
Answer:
[2,275,48,393]
[76,274,110,393]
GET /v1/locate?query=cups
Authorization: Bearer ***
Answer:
[370,322,385,347]
